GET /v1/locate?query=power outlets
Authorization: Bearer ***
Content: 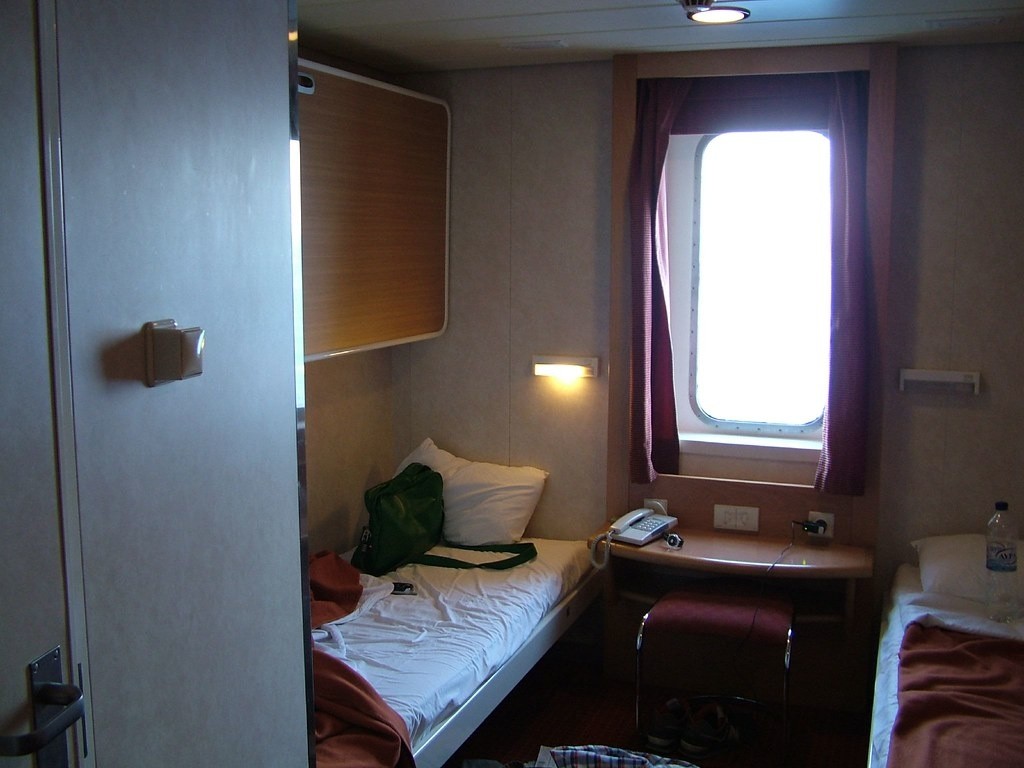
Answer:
[644,499,668,516]
[808,511,834,539]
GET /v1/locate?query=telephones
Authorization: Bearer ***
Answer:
[605,507,679,546]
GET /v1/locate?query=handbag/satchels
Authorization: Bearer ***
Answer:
[350,462,537,578]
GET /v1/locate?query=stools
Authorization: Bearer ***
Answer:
[629,586,795,768]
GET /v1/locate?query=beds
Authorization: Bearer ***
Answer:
[304,535,599,768]
[864,565,1024,768]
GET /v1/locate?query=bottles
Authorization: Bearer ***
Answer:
[985,501,1019,623]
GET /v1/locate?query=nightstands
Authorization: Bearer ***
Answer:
[593,525,876,734]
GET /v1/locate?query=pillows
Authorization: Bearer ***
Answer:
[394,438,548,545]
[909,533,1024,608]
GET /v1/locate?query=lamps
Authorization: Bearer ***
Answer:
[530,355,600,378]
[898,367,982,397]
